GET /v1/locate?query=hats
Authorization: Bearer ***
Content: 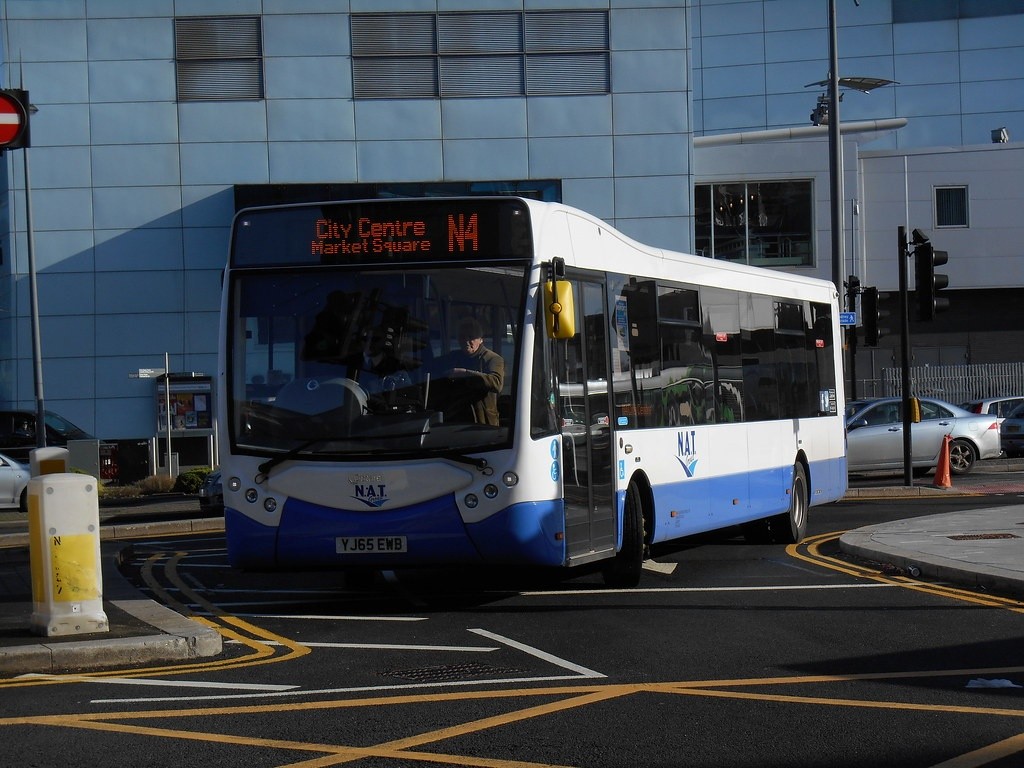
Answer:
[458,317,483,342]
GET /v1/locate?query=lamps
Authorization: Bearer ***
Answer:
[991,126,1010,144]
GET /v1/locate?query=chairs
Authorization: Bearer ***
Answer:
[868,411,898,427]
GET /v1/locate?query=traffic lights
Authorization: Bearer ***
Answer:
[914,242,950,323]
[862,287,891,347]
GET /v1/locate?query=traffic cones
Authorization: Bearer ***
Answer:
[928,437,953,490]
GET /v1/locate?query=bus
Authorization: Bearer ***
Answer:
[212,195,846,594]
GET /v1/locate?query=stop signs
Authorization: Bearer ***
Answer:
[0,89,27,151]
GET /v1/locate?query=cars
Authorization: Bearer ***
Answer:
[846,395,1002,476]
[962,396,1024,457]
[0,408,106,468]
[0,453,32,514]
[199,467,226,517]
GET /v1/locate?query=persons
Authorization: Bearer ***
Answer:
[340,320,406,400]
[14,419,31,436]
[425,316,504,427]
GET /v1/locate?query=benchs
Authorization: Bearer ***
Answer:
[921,411,947,420]
